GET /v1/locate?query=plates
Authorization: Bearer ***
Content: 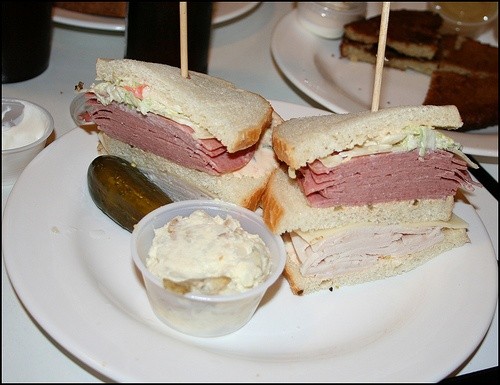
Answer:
[49,1,264,36]
[0,97,500,383]
[268,0,500,160]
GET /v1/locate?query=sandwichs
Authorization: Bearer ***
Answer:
[422,33,500,132]
[262,104,483,296]
[83,57,285,213]
[340,9,445,76]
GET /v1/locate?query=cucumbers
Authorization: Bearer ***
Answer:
[87,155,175,235]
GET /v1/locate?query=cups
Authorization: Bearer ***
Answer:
[0,0,56,84]
[121,1,216,75]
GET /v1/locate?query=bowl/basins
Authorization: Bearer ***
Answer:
[131,200,287,336]
[294,1,368,41]
[0,97,55,184]
[426,1,500,41]
